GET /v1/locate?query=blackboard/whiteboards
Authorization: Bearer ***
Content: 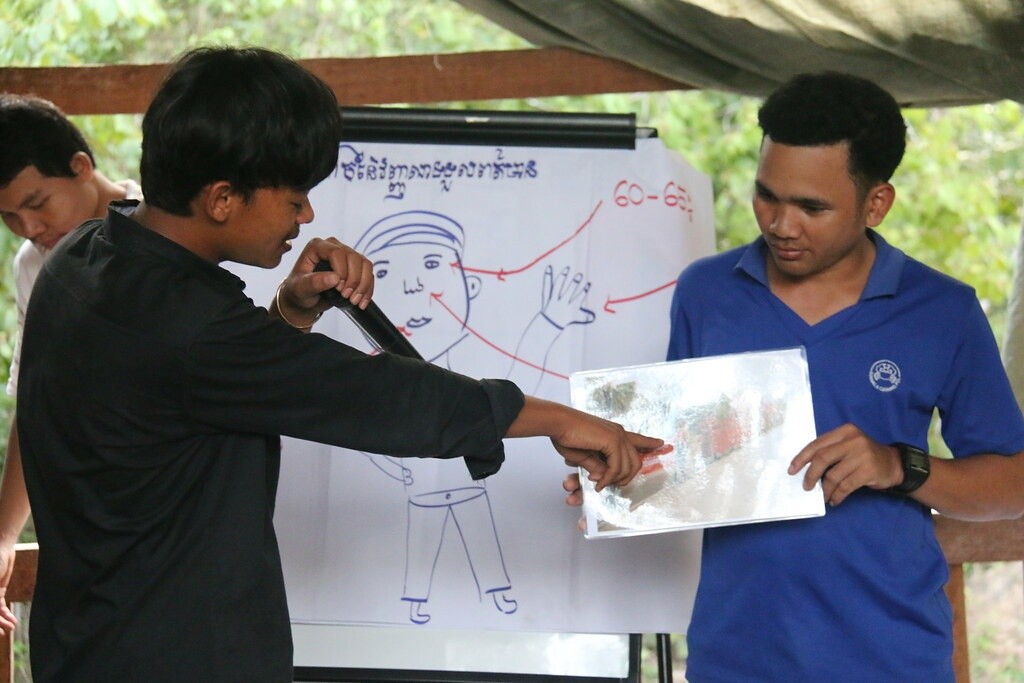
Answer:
[568,345,826,540]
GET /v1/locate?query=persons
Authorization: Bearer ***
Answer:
[16,45,663,683]
[933,514,1024,564]
[0,94,144,635]
[563,70,1024,683]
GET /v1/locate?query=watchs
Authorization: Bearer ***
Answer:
[885,442,931,495]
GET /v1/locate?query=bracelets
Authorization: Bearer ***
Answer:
[276,279,323,328]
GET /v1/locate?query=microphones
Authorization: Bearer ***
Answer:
[311,259,426,361]
